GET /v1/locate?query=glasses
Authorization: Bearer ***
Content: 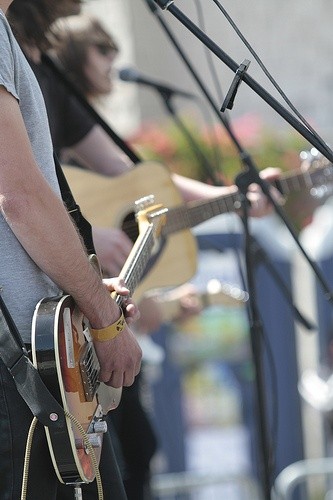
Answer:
[86,38,116,54]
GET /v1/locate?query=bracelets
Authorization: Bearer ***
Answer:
[88,306,127,343]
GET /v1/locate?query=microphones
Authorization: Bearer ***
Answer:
[118,68,191,97]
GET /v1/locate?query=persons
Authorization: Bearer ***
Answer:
[0,0,288,500]
[46,18,206,500]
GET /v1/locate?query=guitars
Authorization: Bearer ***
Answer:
[59,161,333,307]
[130,278,250,368]
[30,192,170,484]
